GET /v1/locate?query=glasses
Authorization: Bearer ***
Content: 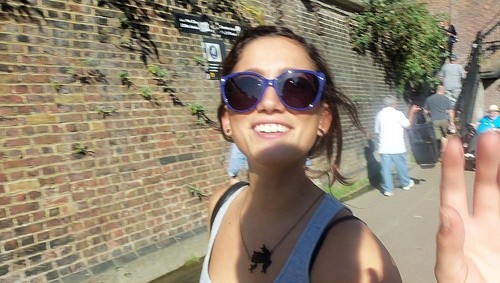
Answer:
[220,70,325,112]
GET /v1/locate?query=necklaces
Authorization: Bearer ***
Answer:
[239,190,324,274]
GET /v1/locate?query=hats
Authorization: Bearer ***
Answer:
[485,105,500,112]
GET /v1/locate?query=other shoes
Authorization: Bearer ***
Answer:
[383,192,394,196]
[403,180,415,189]
[464,153,474,158]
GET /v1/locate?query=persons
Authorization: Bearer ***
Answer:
[374,95,421,197]
[464,105,500,157]
[442,21,457,55]
[423,85,456,161]
[198,24,500,283]
[438,54,467,105]
